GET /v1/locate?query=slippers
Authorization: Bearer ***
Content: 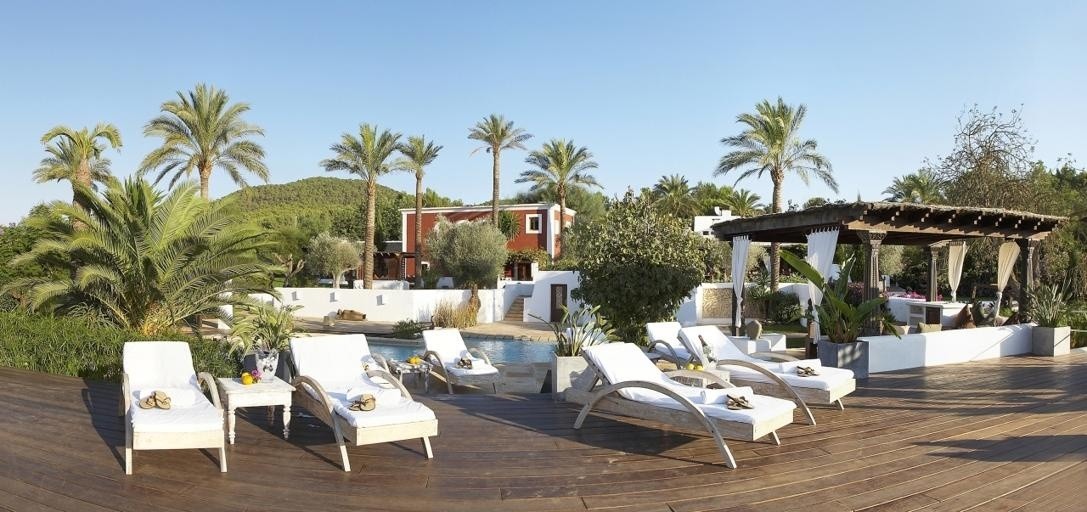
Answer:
[797,366,819,375]
[140,396,156,409]
[727,394,753,408]
[360,394,375,411]
[728,399,741,410]
[155,391,171,409]
[456,358,465,368]
[349,400,360,411]
[465,359,472,369]
[798,369,808,376]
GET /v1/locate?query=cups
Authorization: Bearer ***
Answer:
[255,352,278,382]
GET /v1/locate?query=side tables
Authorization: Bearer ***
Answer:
[217,373,296,446]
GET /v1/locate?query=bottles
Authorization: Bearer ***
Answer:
[698,333,716,362]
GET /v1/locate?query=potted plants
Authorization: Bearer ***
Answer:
[226,304,304,383]
[1022,272,1076,358]
[526,305,625,393]
[777,250,901,381]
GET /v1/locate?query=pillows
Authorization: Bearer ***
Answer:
[881,301,1030,335]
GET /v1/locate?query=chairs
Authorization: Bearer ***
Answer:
[282,328,499,473]
[119,341,228,474]
